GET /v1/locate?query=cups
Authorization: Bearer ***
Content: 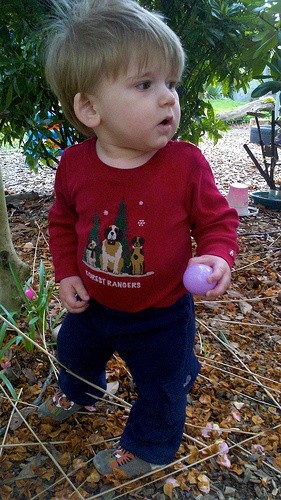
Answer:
[268,188,280,200]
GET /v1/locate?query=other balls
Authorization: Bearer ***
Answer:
[184,265,215,297]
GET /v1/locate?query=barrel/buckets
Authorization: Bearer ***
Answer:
[227,183,259,217]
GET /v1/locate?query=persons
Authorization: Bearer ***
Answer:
[34,0,240,481]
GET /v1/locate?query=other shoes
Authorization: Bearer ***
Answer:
[92,444,167,479]
[37,390,83,424]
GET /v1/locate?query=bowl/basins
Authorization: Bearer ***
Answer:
[250,191,281,210]
